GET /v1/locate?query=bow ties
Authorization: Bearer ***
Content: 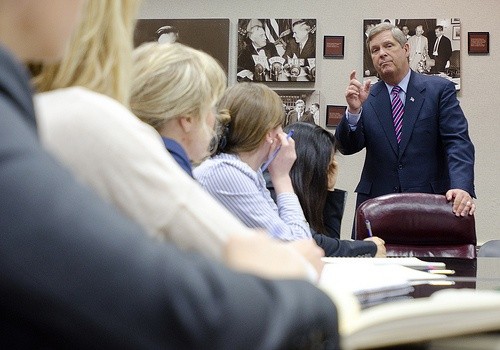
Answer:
[257,46,266,52]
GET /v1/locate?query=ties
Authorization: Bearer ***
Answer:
[435,39,439,52]
[300,112,301,119]
[390,86,405,144]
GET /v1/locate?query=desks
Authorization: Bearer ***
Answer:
[356,258,500,350]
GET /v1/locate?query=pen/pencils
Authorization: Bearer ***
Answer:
[364,219,373,237]
[261,129,295,172]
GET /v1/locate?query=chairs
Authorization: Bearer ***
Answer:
[449,50,460,67]
[350,192,477,257]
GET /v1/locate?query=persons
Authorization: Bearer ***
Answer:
[238,26,280,72]
[191,83,313,240]
[283,20,315,58]
[26,0,324,284]
[428,25,451,74]
[257,121,387,258]
[1,0,343,350]
[288,99,315,126]
[155,24,179,43]
[334,22,478,240]
[311,103,319,126]
[126,42,227,181]
[401,25,411,41]
[406,25,428,75]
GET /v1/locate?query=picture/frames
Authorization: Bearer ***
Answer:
[452,26,460,40]
[323,36,345,58]
[326,105,347,127]
[451,17,460,24]
[468,32,489,54]
